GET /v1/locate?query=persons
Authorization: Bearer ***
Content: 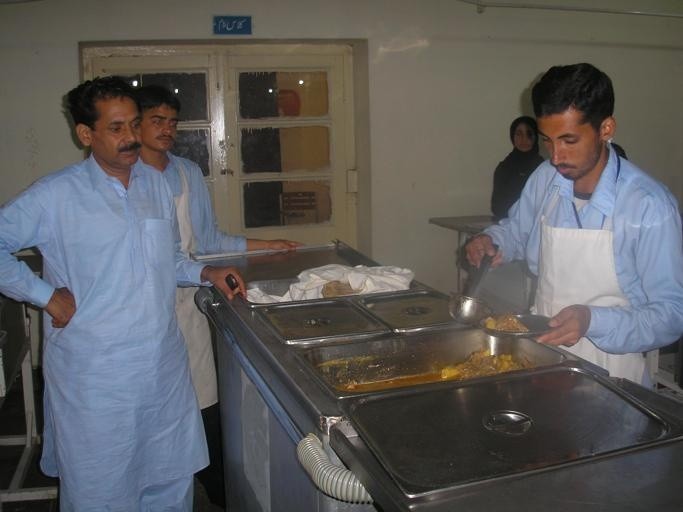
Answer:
[137,84,306,508]
[0,74,247,512]
[491,117,545,218]
[465,63,683,391]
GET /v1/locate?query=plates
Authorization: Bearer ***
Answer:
[479,314,552,339]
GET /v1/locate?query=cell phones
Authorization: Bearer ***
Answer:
[225,274,239,290]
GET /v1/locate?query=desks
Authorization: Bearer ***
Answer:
[428,215,498,295]
